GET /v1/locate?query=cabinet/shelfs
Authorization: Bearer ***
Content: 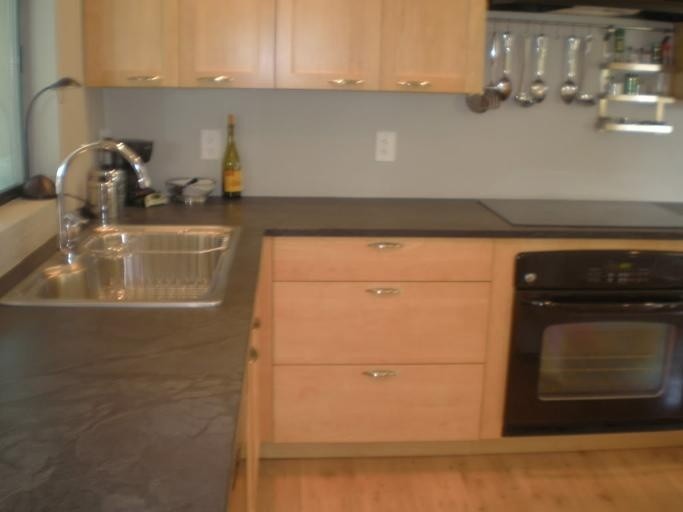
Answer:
[278,0,488,97]
[599,57,679,135]
[85,0,279,92]
[273,236,486,451]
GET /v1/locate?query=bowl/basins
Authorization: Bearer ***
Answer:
[167,175,216,208]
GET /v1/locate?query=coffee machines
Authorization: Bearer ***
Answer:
[97,135,154,211]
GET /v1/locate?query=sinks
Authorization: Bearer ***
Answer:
[67,225,242,253]
[1,250,233,308]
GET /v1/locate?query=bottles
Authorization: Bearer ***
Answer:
[89,165,127,218]
[613,28,670,66]
[220,114,242,201]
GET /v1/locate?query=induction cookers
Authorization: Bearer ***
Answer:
[472,198,681,295]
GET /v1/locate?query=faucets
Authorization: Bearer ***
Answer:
[54,139,151,250]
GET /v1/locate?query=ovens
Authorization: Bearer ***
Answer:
[497,296,682,437]
